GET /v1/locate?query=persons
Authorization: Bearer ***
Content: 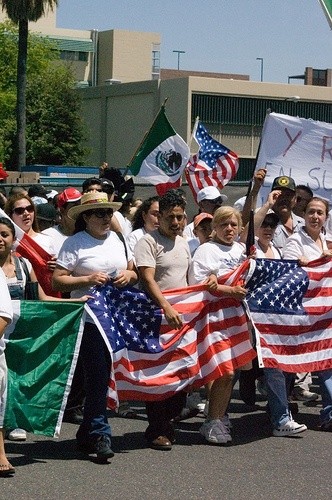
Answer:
[0,161,332,474]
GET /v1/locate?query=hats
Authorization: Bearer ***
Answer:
[46,189,58,199]
[28,186,52,197]
[254,207,277,216]
[233,196,246,212]
[100,178,115,189]
[57,188,82,207]
[67,191,122,222]
[193,212,213,229]
[30,196,48,209]
[196,186,228,204]
[34,202,56,222]
[272,176,296,193]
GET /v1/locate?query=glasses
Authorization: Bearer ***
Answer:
[260,222,278,230]
[207,198,224,205]
[11,204,35,215]
[103,188,114,194]
[296,195,311,203]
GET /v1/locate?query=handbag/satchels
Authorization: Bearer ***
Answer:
[19,257,40,300]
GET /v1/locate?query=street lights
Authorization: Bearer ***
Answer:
[172,50,186,70]
[256,57,263,81]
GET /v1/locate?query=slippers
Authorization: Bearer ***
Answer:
[0,463,16,474]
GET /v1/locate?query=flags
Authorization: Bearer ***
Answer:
[83,245,253,411]
[4,299,88,436]
[129,112,240,204]
[242,258,332,373]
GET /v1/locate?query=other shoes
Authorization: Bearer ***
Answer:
[145,383,332,450]
[95,439,114,461]
[61,407,84,425]
[9,428,26,440]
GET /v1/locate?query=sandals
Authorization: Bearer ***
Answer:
[118,401,137,418]
